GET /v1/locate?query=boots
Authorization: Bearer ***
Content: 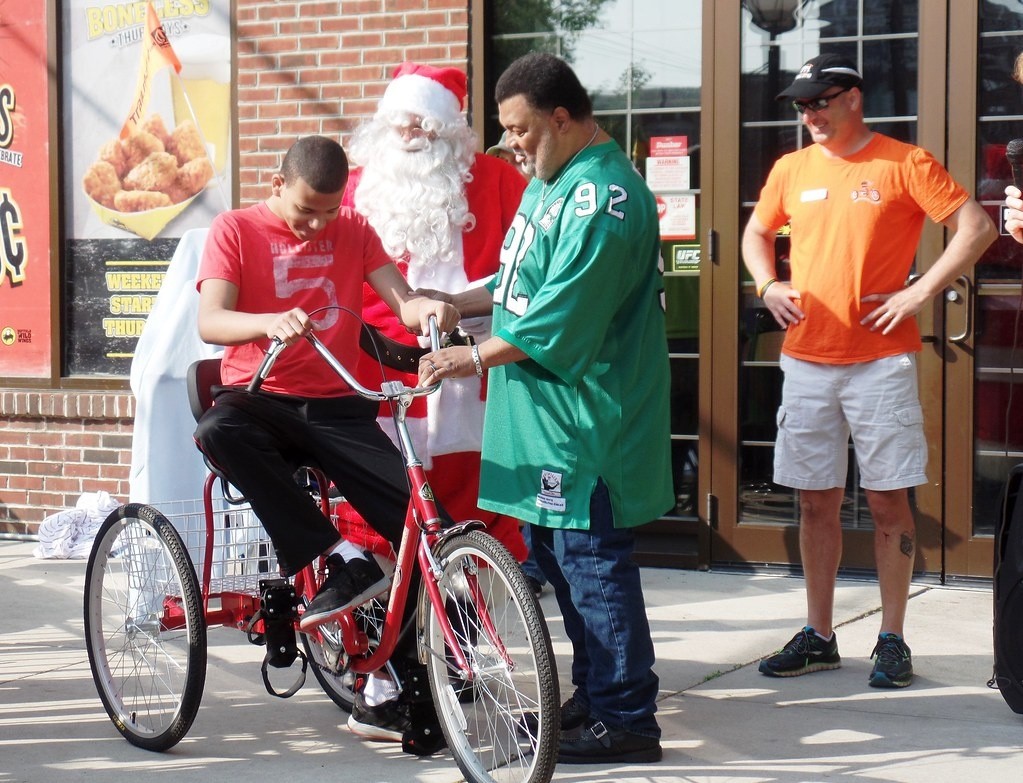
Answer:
[445,598,483,703]
[346,598,387,695]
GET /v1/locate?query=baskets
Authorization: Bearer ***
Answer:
[116,495,340,624]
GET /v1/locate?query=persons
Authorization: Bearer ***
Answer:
[741,56,997,689]
[658,196,749,518]
[196,136,461,743]
[397,55,675,765]
[486,132,547,600]
[342,63,528,702]
[1004,54,1023,243]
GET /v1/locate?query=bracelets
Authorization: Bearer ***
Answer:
[758,277,780,299]
[472,345,484,377]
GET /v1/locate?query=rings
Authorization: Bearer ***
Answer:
[431,364,437,371]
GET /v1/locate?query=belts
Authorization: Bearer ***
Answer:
[359,323,476,375]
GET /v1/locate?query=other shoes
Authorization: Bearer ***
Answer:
[509,575,542,601]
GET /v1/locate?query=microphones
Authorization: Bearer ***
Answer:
[1005,139,1023,201]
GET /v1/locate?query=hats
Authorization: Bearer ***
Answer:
[485,131,515,156]
[383,61,468,124]
[774,53,864,101]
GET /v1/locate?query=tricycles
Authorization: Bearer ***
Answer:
[83,303,560,783]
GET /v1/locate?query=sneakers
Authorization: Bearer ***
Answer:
[347,685,412,742]
[869,633,913,688]
[530,718,662,764]
[299,549,391,633]
[518,691,590,738]
[758,627,842,677]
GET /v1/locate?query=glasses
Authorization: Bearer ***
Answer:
[792,85,862,114]
